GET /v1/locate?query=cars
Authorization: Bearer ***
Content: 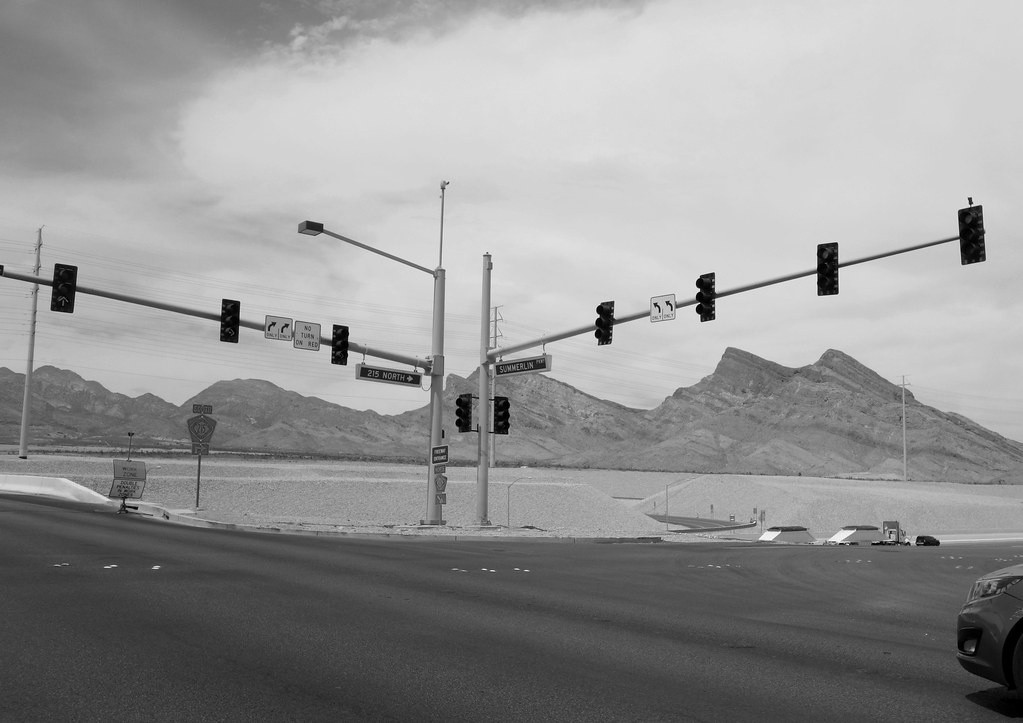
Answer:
[956,563,1023,704]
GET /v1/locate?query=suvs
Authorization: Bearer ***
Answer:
[916,536,940,546]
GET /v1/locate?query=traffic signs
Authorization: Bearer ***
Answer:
[356,364,422,388]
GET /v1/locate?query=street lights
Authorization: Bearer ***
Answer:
[298,220,445,524]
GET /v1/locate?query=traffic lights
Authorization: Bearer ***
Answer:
[220,299,240,343]
[696,272,715,322]
[958,205,986,265]
[595,301,614,346]
[50,263,78,313]
[494,396,510,434]
[331,324,349,365]
[456,393,473,432]
[817,242,839,296]
